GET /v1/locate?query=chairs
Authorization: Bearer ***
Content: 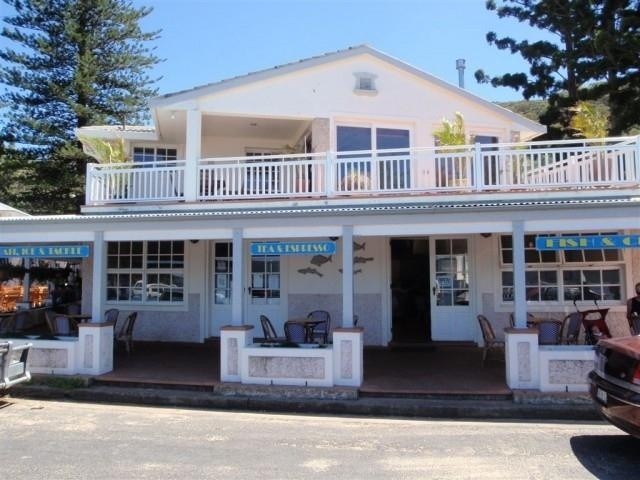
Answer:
[478,309,585,370]
[260,310,359,345]
[1,302,136,361]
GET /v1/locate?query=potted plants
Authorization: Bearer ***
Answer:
[566,99,613,188]
[430,111,470,193]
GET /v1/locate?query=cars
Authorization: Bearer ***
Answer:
[133,280,183,300]
[587,334,639,439]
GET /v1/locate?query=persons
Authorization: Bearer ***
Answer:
[626,281,640,338]
